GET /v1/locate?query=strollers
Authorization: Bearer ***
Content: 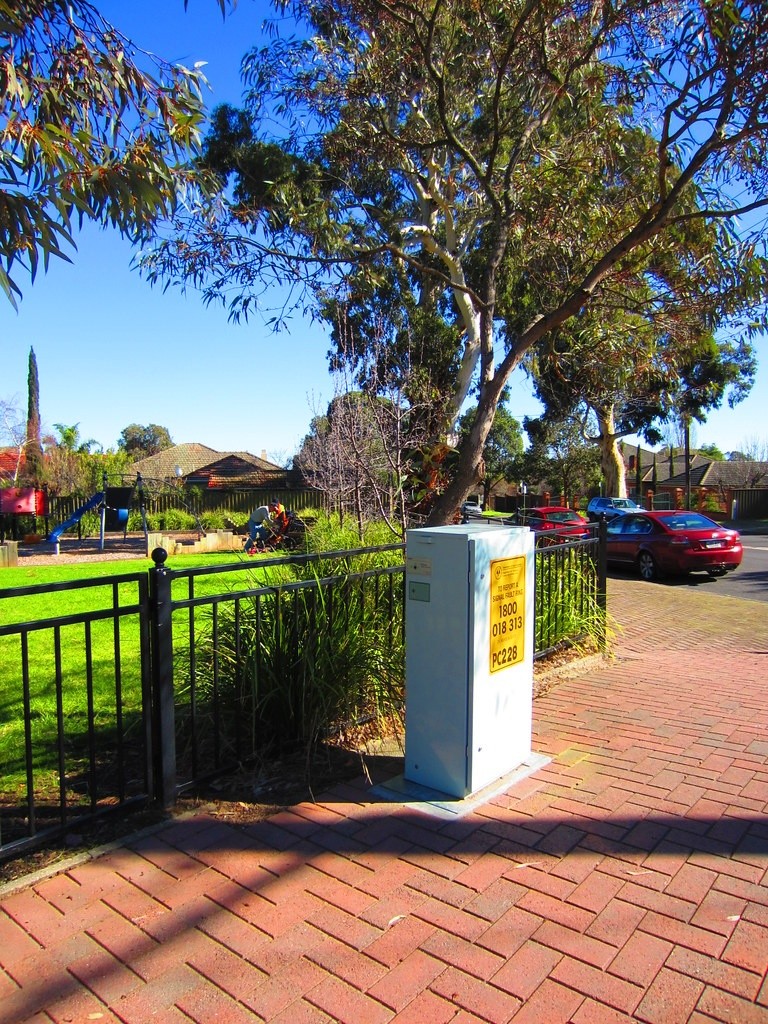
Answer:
[269,512,297,551]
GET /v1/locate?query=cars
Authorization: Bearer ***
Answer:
[585,509,744,584]
[507,507,589,557]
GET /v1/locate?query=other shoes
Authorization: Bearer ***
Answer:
[245,549,248,551]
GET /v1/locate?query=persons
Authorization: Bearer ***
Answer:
[243,503,277,551]
[272,498,285,519]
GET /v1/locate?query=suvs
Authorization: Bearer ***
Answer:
[461,501,483,518]
[586,497,648,523]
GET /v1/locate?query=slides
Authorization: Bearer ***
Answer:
[44,491,105,545]
